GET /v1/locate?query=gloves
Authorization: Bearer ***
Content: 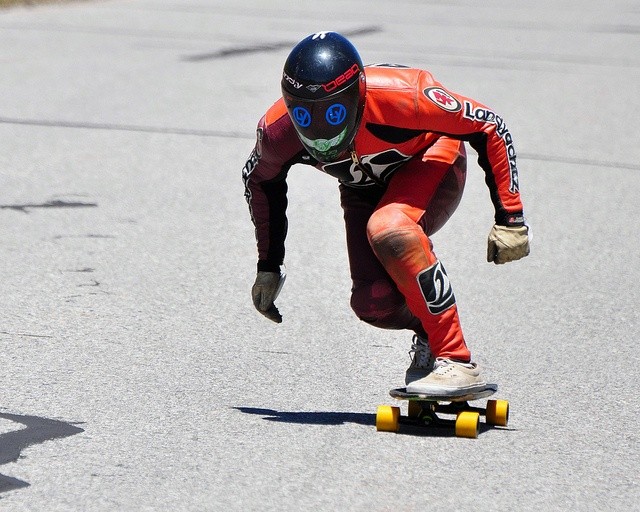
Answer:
[251,265,286,324]
[487,212,529,264]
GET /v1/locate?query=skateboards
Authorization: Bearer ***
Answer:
[376,383,509,438]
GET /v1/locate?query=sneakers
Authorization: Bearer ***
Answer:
[406,355,486,397]
[406,335,433,384]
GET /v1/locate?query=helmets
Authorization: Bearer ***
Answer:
[281,31,365,162]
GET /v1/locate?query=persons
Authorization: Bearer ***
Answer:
[241,31,532,402]
